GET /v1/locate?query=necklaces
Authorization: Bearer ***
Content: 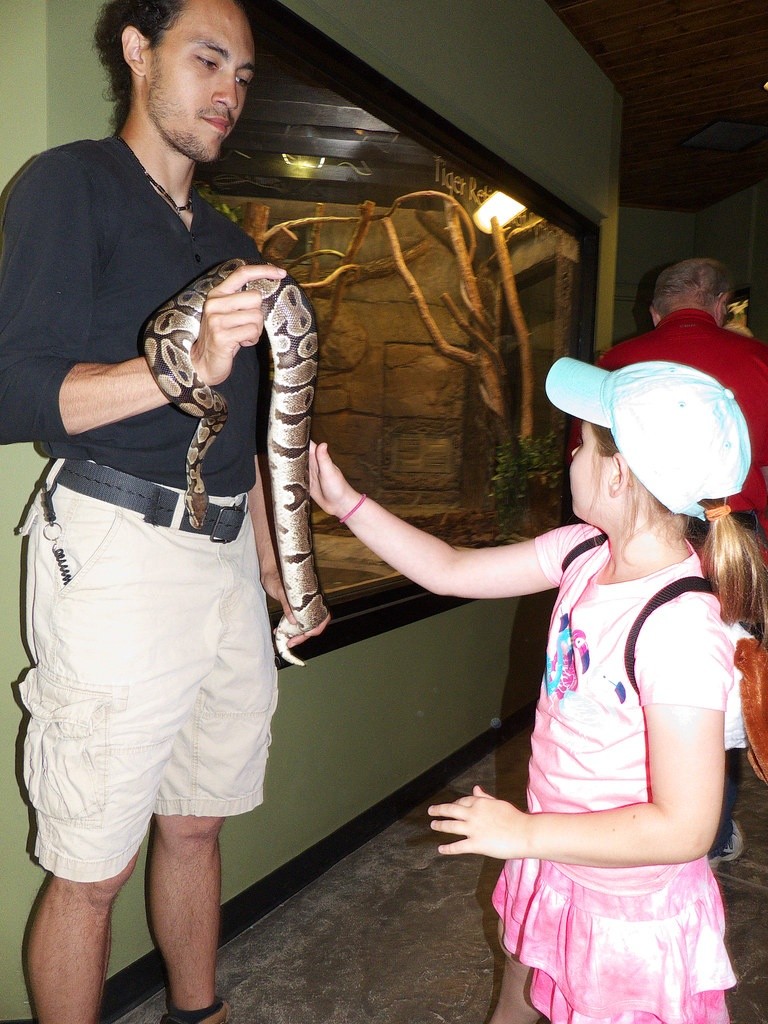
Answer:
[112,134,194,221]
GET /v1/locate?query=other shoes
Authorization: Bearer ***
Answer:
[161,997,230,1024]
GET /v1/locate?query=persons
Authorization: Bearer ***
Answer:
[1,0,331,1024]
[307,354,768,1023]
[597,255,768,873]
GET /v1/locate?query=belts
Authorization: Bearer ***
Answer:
[57,462,246,544]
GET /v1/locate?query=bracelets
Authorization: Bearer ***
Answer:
[340,493,366,524]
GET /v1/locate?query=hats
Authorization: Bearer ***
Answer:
[545,354,752,521]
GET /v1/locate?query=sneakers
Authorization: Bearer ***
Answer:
[708,821,745,869]
